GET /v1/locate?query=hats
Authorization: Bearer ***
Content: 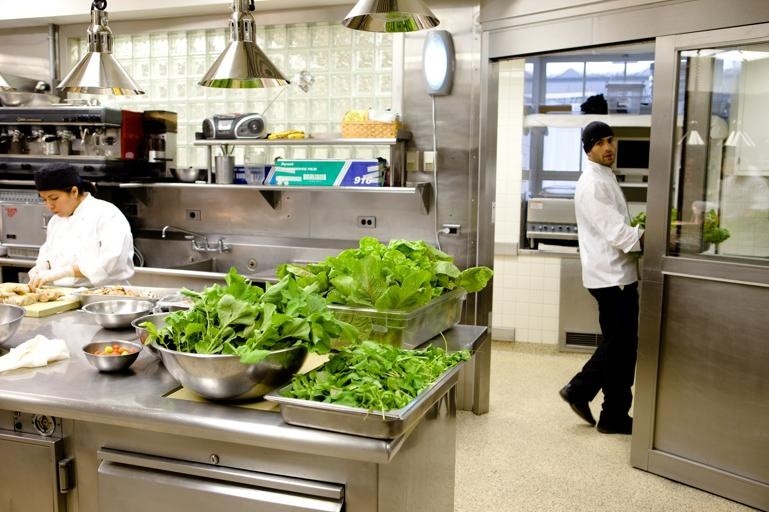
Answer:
[34,164,81,191]
[582,122,614,153]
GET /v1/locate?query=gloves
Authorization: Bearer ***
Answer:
[28,262,73,291]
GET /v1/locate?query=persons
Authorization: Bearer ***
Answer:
[558,120,644,435]
[27,164,134,294]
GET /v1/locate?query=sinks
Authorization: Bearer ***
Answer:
[172,244,296,279]
[133,237,213,269]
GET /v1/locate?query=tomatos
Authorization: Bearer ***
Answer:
[95,344,137,356]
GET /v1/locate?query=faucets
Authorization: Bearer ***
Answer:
[160,224,230,254]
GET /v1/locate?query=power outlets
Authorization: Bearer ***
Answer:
[439,222,461,237]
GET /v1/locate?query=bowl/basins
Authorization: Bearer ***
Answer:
[131,310,181,362]
[148,335,309,407]
[80,341,142,374]
[0,91,34,106]
[77,299,154,329]
[0,304,26,347]
[168,167,208,183]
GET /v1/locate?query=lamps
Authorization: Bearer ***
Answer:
[339,0,441,38]
[49,0,146,98]
[418,28,457,97]
[720,57,755,148]
[199,0,292,90]
[674,48,707,147]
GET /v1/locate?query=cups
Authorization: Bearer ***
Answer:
[243,150,265,185]
[215,155,234,184]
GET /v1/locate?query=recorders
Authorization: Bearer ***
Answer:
[202,113,267,139]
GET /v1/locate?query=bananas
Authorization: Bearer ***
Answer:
[267,130,305,140]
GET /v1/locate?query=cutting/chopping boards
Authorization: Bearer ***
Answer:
[0,281,83,319]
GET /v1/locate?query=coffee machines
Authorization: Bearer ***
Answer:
[0,104,179,180]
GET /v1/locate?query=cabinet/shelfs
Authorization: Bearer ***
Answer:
[138,131,432,219]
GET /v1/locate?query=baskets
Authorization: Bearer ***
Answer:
[343,122,401,139]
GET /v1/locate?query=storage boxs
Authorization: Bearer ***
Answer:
[603,80,647,115]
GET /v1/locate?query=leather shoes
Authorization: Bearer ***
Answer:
[598,414,633,434]
[560,383,594,426]
